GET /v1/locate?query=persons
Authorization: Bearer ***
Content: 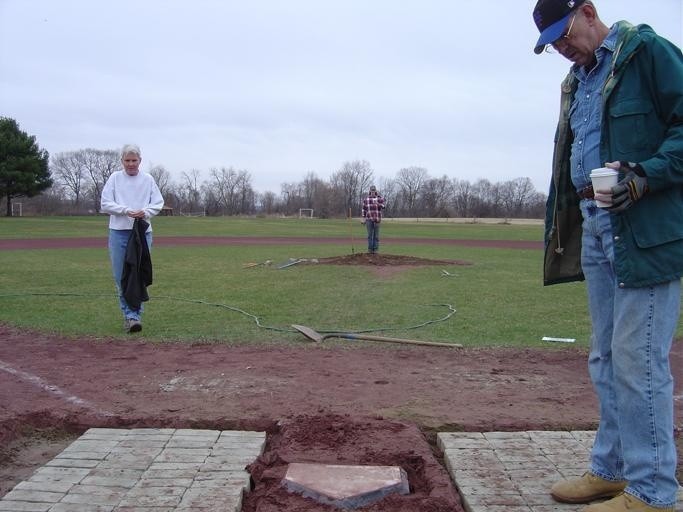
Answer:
[361,186,385,254]
[100,143,165,332]
[532,0,683,512]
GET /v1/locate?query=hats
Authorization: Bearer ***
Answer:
[533,1,586,54]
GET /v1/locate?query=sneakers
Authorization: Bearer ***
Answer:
[123,319,141,332]
[551,472,626,504]
[584,491,674,511]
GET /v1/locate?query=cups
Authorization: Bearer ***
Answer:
[587,167,619,209]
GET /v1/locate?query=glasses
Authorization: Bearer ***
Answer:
[545,14,575,52]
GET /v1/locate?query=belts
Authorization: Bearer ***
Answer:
[576,186,596,200]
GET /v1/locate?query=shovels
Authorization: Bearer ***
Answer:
[290,324,462,348]
